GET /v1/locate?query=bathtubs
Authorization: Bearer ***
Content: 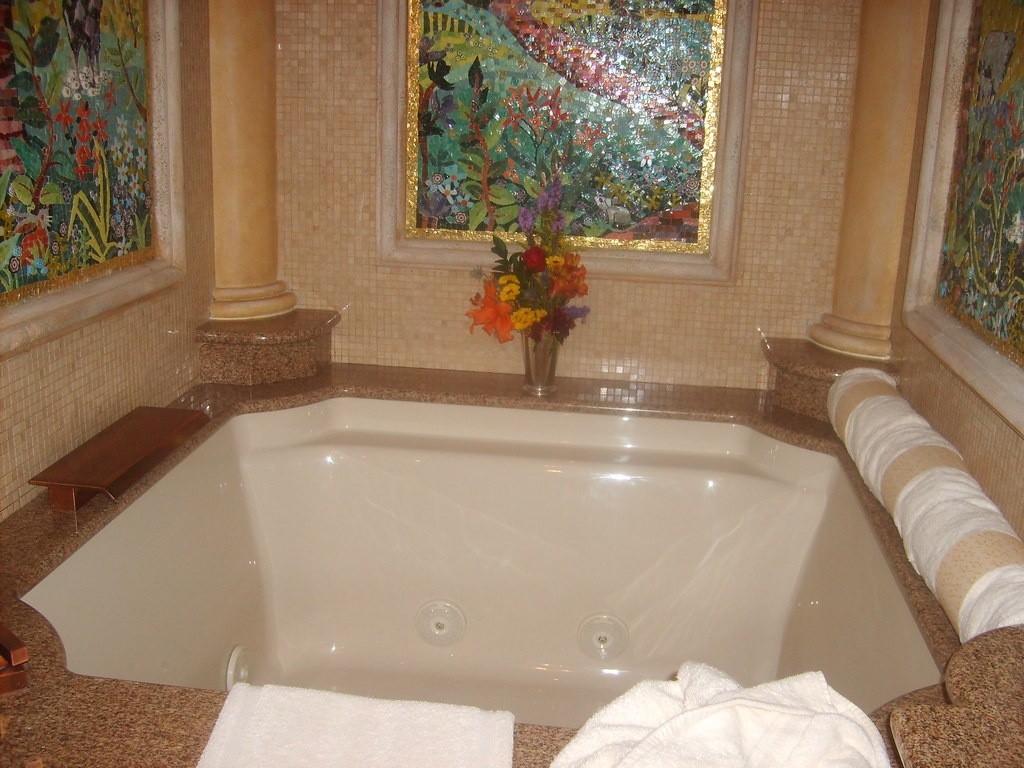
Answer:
[0,363,1024,768]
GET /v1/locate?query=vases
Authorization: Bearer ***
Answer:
[520,330,557,395]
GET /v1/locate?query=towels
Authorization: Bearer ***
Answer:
[195,681,515,768]
[547,660,891,768]
[826,367,1024,644]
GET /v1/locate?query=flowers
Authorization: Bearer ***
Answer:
[464,174,591,346]
